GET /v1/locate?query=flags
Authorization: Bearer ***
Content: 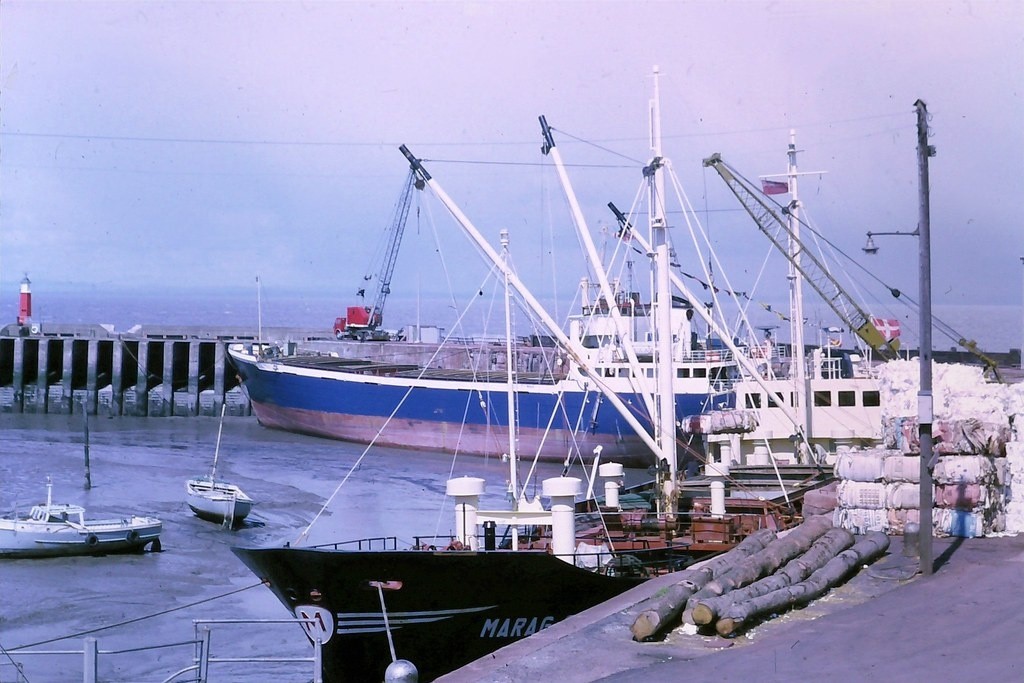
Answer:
[762,180,788,194]
[870,319,901,344]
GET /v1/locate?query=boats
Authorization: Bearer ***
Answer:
[227,258,902,471]
[185,403,254,523]
[0,474,163,559]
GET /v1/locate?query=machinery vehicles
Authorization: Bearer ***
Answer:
[332,168,419,342]
[701,150,1017,387]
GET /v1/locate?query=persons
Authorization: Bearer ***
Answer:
[760,331,775,356]
[809,443,827,464]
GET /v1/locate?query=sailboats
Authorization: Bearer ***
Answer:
[231,62,839,683]
